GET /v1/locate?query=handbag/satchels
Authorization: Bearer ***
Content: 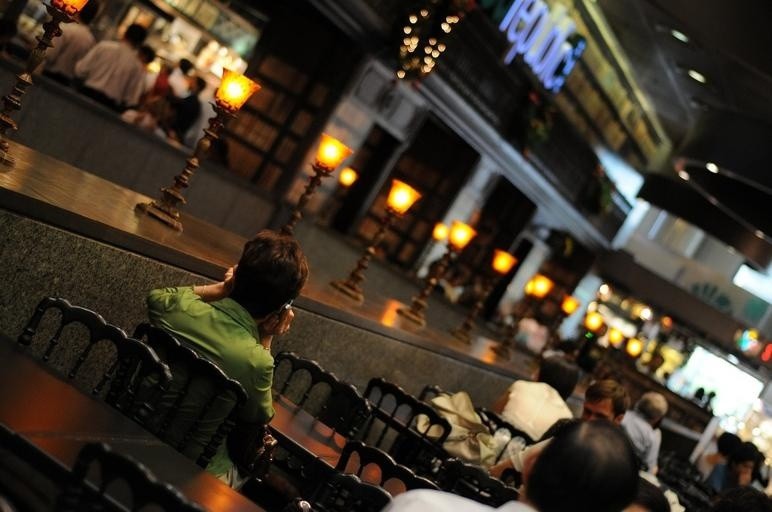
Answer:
[414,390,498,471]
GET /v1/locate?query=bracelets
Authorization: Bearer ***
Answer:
[201,284,207,303]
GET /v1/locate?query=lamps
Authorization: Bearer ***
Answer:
[134,68,645,381]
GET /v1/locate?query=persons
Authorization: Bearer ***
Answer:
[43,0,207,144]
[490,379,631,480]
[509,307,554,359]
[383,417,639,510]
[702,440,763,494]
[493,354,581,440]
[135,228,309,492]
[696,431,741,483]
[620,391,668,474]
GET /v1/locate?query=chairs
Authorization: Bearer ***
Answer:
[17,295,720,512]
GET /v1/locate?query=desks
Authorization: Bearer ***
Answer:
[0,334,261,511]
